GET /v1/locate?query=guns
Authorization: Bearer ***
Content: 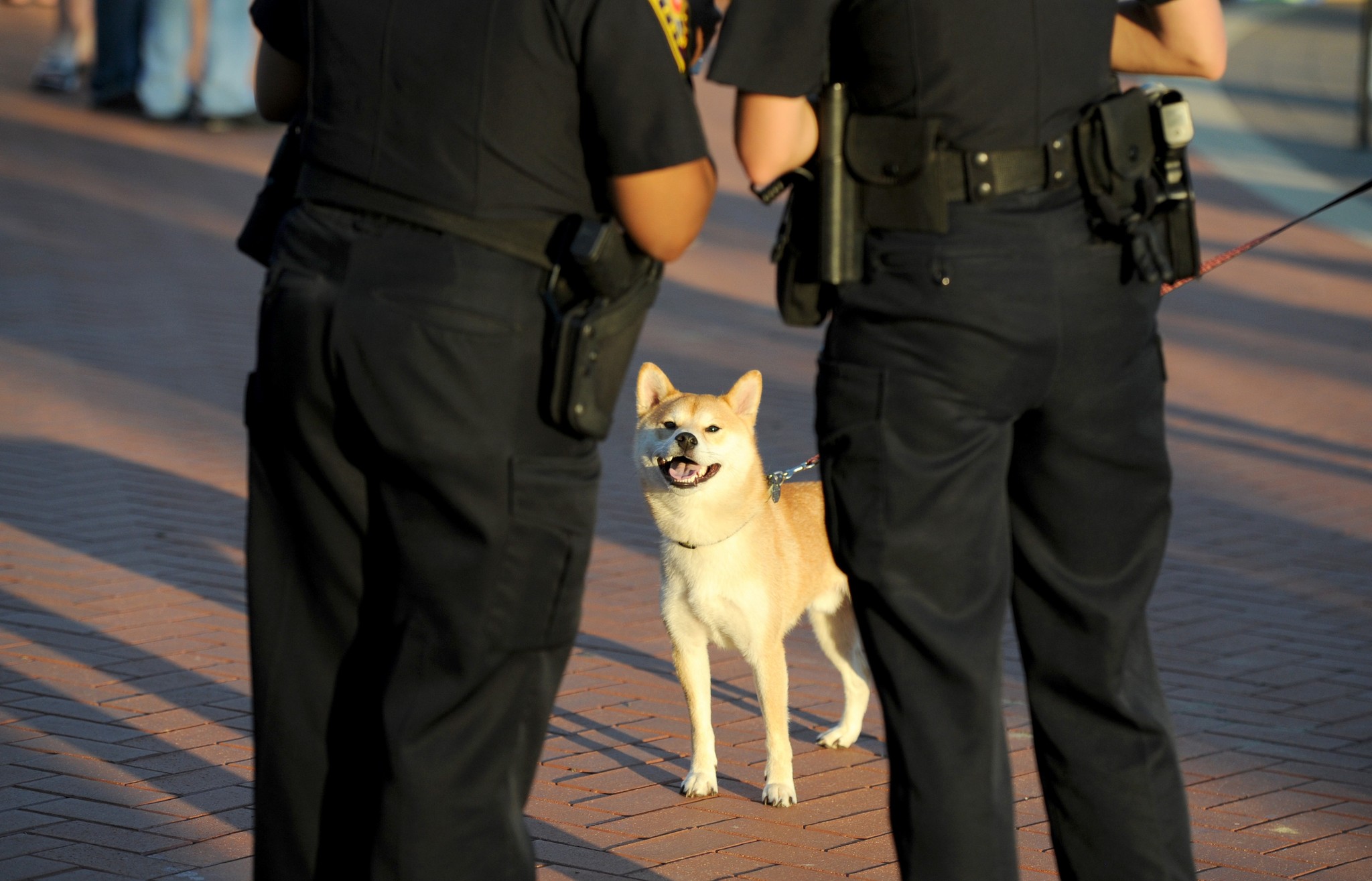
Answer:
[549,207,665,442]
[1156,87,1199,276]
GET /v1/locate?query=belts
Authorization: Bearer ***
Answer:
[296,166,562,269]
[937,150,1082,202]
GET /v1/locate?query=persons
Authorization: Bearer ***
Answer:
[708,0,1229,881]
[235,0,719,881]
[56,0,257,136]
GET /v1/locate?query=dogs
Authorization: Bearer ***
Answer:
[633,360,872,808]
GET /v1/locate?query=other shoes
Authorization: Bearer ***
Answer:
[36,42,81,92]
[202,111,272,132]
